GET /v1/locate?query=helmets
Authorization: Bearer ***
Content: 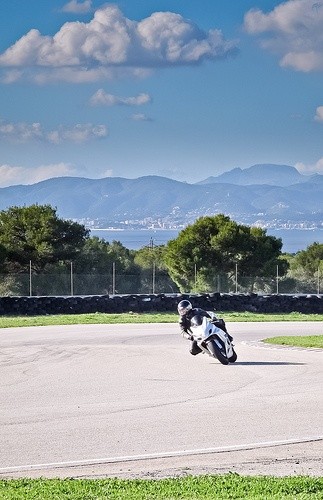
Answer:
[177,300,192,315]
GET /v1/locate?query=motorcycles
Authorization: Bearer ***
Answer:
[185,315,238,365]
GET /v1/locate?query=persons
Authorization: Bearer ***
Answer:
[178,300,233,356]
